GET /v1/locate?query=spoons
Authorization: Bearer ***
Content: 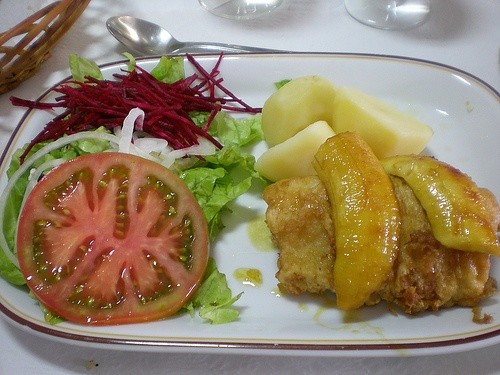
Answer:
[107,15,281,55]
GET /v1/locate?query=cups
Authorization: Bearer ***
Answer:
[345,0,432,32]
[198,0,283,22]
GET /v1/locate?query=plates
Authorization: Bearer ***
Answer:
[0,50,500,357]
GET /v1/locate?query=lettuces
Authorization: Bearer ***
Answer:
[0,50,294,325]
